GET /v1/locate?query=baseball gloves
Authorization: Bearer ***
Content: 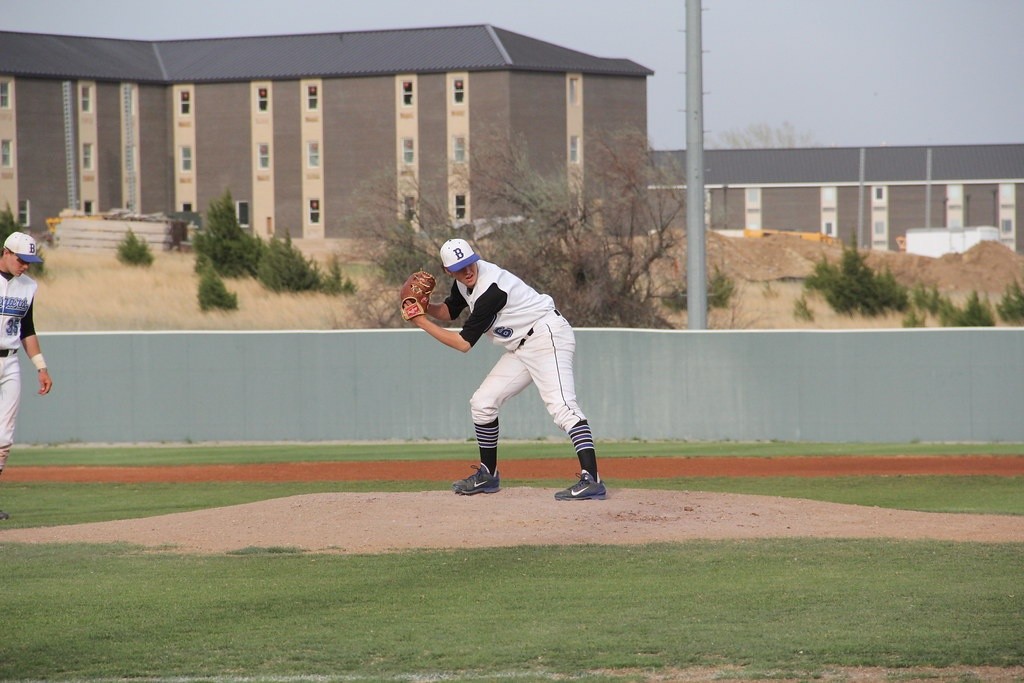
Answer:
[400,267,437,321]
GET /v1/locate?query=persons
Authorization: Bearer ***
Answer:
[400,239,606,501]
[0,232,52,519]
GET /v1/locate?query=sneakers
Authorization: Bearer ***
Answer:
[452,465,500,494]
[554,473,607,501]
[0,510,9,520]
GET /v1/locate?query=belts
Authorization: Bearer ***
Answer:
[0,350,17,357]
[520,310,561,345]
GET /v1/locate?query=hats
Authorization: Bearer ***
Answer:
[3,232,44,263]
[440,238,480,272]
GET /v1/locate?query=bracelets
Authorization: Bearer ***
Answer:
[38,368,47,373]
